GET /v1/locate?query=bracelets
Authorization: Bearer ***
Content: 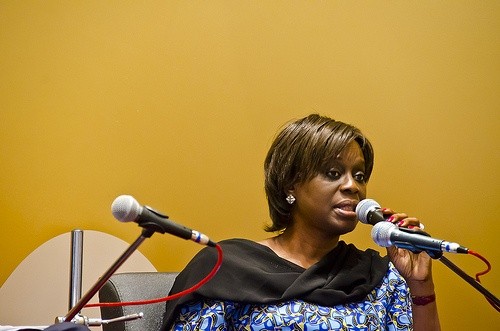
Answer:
[411,293,436,306]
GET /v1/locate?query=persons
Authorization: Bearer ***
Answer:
[159,113,444,331]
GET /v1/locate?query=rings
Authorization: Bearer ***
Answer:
[419,222,425,230]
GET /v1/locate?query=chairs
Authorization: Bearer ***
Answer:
[98,272,180,330]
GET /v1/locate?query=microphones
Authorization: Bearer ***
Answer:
[111,194,216,248]
[355,198,443,259]
[371,221,469,254]
